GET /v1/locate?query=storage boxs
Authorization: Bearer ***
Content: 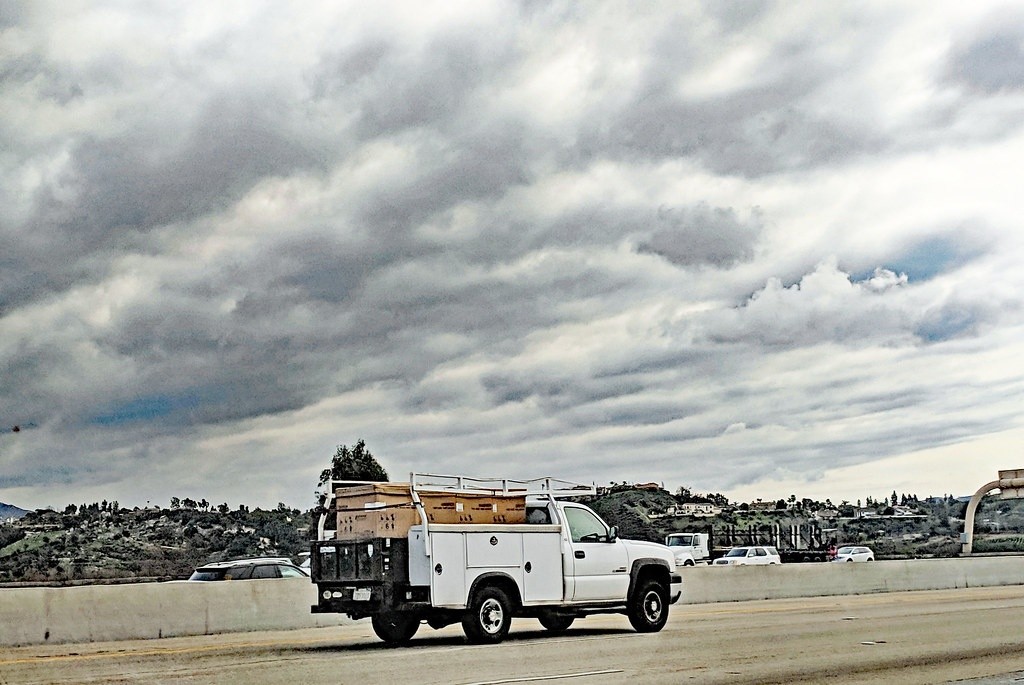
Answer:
[308,485,527,579]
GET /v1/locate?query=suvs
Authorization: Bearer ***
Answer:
[189,560,309,581]
[832,547,874,563]
[712,547,782,564]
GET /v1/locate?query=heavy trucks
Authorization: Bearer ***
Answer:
[663,526,837,563]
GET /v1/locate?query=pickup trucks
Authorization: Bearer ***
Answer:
[309,473,682,645]
